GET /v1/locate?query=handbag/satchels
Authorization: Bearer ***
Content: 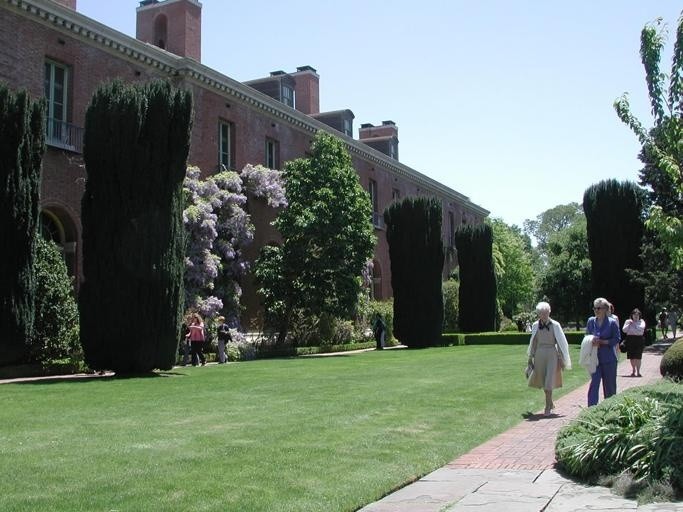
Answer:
[525,363,534,378]
[620,340,627,353]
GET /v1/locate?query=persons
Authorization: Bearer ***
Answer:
[178,312,207,367]
[658,307,669,340]
[524,320,532,332]
[621,308,646,377]
[212,315,230,364]
[585,297,623,410]
[379,322,386,349]
[526,301,572,420]
[516,316,524,331]
[608,303,619,354]
[668,307,676,340]
[374,313,382,349]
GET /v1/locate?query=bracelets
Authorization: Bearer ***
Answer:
[224,331,227,335]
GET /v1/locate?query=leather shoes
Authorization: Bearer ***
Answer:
[543,408,552,418]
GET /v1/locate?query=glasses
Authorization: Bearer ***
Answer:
[592,307,605,310]
